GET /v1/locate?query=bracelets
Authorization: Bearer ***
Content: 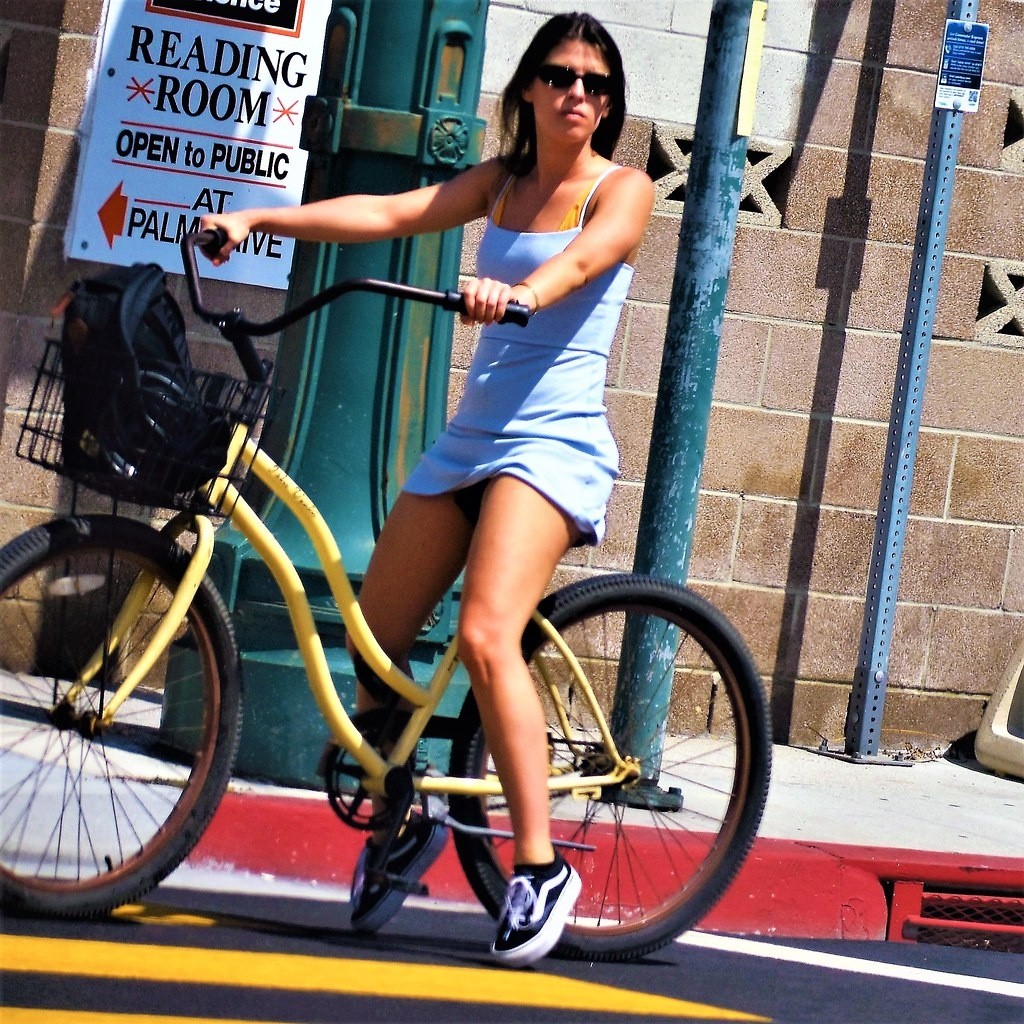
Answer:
[513,282,540,316]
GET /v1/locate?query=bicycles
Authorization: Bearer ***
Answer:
[0,228,772,965]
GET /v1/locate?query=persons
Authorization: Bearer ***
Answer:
[193,13,656,965]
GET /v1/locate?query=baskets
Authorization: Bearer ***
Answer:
[16,338,285,518]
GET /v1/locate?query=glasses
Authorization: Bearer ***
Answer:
[537,62,618,98]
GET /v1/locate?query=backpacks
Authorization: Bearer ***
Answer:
[57,260,231,503]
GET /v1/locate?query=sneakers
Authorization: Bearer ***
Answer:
[488,841,584,970]
[348,807,449,935]
